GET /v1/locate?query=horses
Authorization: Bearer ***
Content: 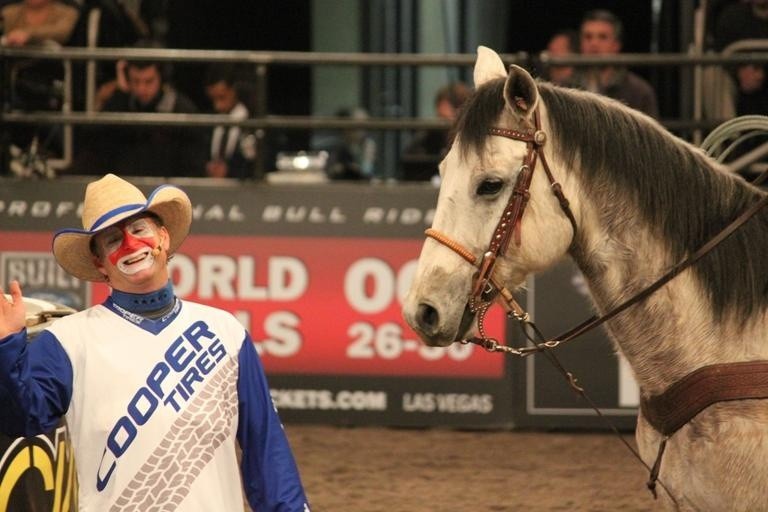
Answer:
[400,43,768,512]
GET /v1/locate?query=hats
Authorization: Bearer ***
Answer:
[51,173,193,281]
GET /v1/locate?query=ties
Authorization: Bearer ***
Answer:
[220,125,230,160]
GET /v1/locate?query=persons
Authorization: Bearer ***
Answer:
[0,170,309,511]
[699,0,768,140]
[0,0,263,185]
[327,107,376,181]
[403,83,472,182]
[547,12,659,121]
